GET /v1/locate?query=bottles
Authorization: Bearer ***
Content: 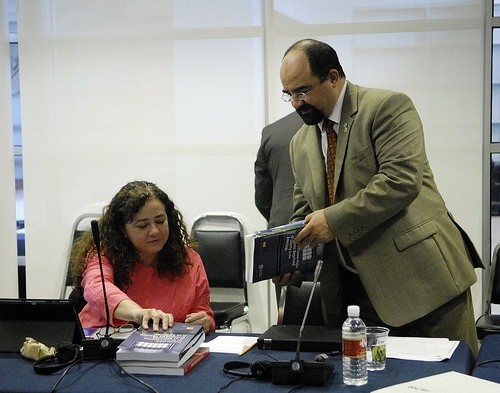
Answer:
[341,305,368,386]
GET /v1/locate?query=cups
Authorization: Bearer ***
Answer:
[366,326,390,371]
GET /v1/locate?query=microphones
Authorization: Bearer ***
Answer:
[272,260,334,388]
[81,221,127,360]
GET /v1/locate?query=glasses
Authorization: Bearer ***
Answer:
[281,75,327,103]
[95,323,140,341]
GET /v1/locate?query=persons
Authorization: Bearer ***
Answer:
[271,38,486,361]
[253,108,328,326]
[68,180,216,335]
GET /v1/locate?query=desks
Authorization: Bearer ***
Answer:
[0,331,472,393]
[470,334,500,384]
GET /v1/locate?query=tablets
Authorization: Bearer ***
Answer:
[0,299,86,322]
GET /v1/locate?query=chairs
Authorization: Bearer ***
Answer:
[189,213,252,337]
[476,241,500,339]
[277,280,323,327]
[61,212,106,299]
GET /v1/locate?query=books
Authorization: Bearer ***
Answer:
[116,316,212,376]
[244,219,323,284]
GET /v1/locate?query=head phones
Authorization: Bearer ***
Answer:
[222,360,272,382]
[33,343,82,375]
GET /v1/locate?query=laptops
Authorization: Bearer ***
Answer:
[257,325,376,352]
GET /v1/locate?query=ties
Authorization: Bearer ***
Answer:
[322,117,338,207]
